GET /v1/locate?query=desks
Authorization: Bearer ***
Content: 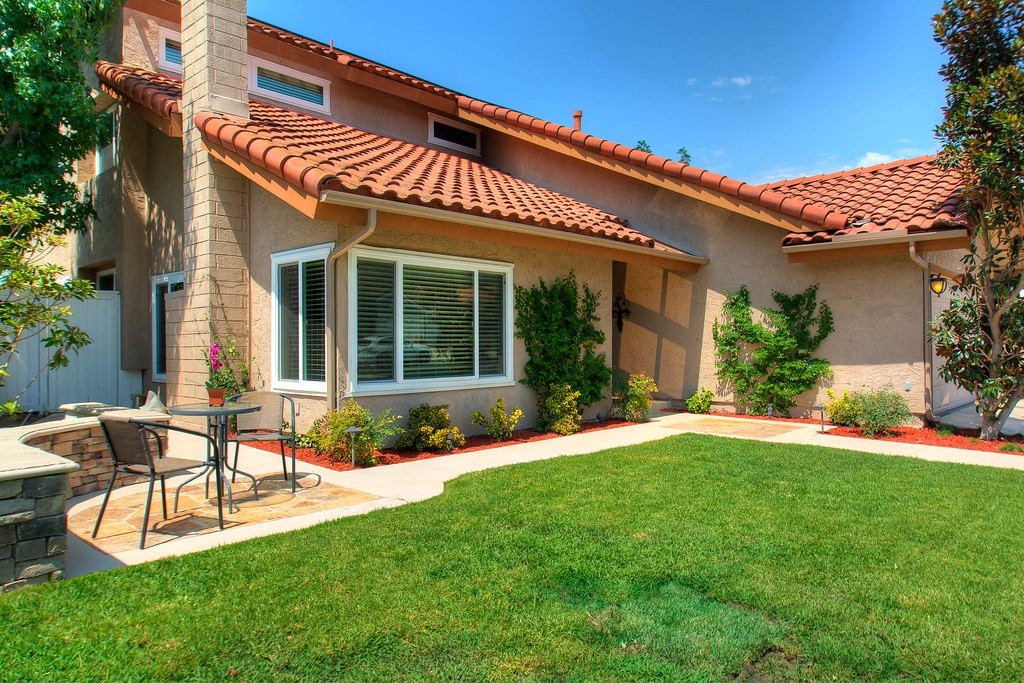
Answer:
[168,402,263,514]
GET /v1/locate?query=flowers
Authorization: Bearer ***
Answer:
[200,343,235,389]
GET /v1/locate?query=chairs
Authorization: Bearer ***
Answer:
[91,416,223,549]
[222,390,296,492]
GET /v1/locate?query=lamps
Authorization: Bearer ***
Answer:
[345,426,363,440]
[929,273,947,297]
[817,403,828,410]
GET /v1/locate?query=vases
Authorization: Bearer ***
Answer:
[207,388,226,407]
